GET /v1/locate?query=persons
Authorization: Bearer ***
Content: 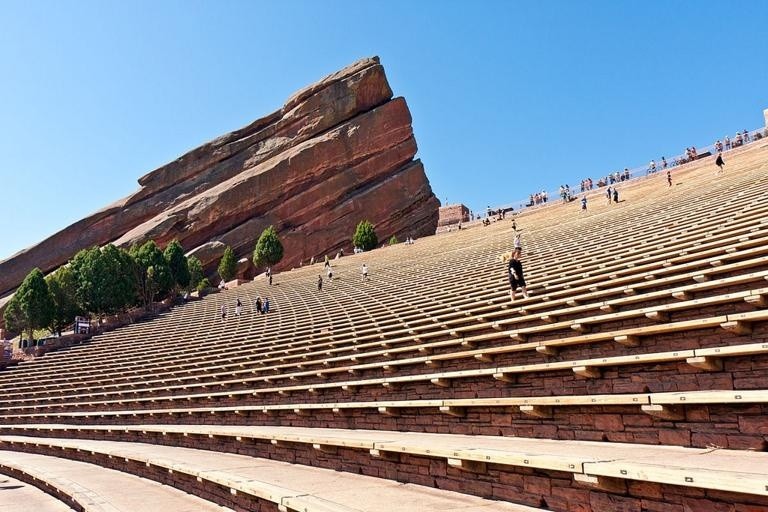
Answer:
[716,152,725,174]
[715,128,761,153]
[307,247,344,292]
[507,250,529,301]
[596,167,629,206]
[580,177,593,192]
[234,298,243,315]
[511,219,517,234]
[255,295,270,315]
[581,195,587,212]
[665,170,672,187]
[646,144,698,173]
[514,234,521,247]
[457,205,506,230]
[559,184,571,205]
[502,246,522,265]
[528,187,547,207]
[221,302,228,319]
[362,263,368,281]
